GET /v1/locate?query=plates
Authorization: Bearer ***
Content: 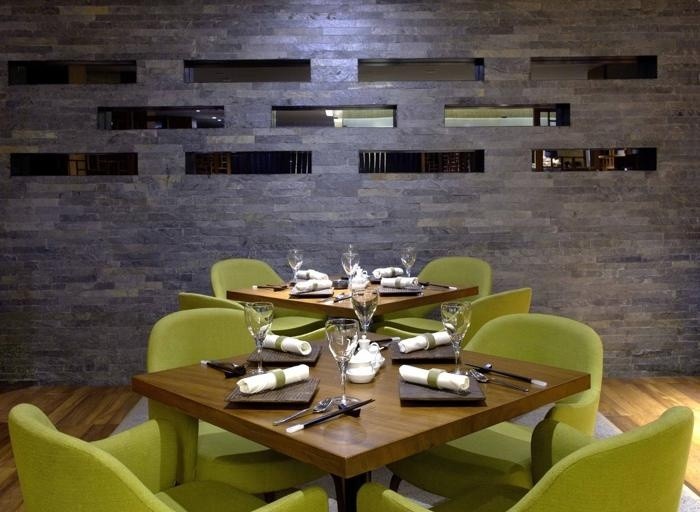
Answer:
[390,339,461,362]
[380,285,422,295]
[289,288,335,298]
[399,369,486,402]
[247,340,322,363]
[223,375,320,405]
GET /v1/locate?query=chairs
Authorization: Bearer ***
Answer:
[357,404,693,512]
[7,402,328,512]
[130,259,603,512]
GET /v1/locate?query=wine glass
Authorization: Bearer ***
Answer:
[287,248,303,282]
[440,302,471,375]
[244,301,274,375]
[351,288,380,340]
[400,246,416,277]
[341,251,361,295]
[325,318,362,407]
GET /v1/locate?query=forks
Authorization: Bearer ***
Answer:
[471,369,529,392]
[273,398,332,425]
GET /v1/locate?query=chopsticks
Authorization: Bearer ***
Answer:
[464,363,548,387]
[370,338,400,342]
[285,399,376,434]
